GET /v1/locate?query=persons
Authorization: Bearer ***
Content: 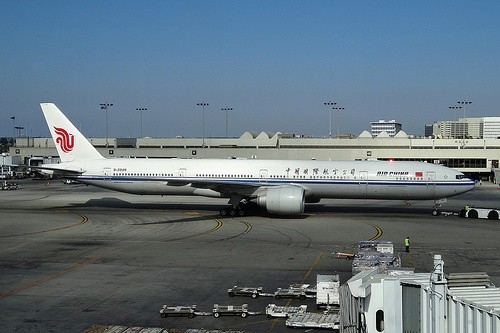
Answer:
[405,237,410,252]
[465,203,471,211]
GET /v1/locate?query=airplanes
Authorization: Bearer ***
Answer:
[2,102,476,218]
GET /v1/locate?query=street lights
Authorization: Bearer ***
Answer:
[197,103,209,149]
[449,107,462,139]
[221,107,233,138]
[332,107,344,139]
[457,101,473,139]
[100,103,114,149]
[136,107,147,137]
[324,103,337,139]
